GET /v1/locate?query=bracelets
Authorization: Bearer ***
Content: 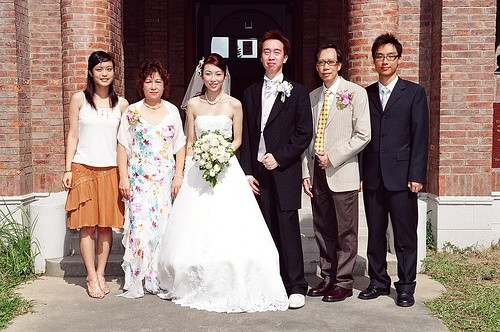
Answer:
[174,174,183,178]
[64,171,71,173]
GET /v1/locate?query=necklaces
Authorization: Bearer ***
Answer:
[205,90,224,104]
[143,101,162,109]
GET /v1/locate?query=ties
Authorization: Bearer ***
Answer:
[265,80,275,99]
[314,90,332,154]
[381,88,390,111]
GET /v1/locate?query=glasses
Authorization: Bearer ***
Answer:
[375,54,399,61]
[316,60,339,66]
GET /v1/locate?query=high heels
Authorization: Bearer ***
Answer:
[98,274,109,295]
[86,275,104,299]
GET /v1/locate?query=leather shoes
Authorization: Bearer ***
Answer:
[322,284,353,302]
[309,280,334,296]
[358,282,390,299]
[289,293,306,308]
[396,291,414,307]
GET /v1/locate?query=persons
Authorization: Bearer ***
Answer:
[62,51,129,298]
[156,53,290,313]
[117,60,188,299]
[301,43,372,301]
[239,31,313,308]
[357,32,429,307]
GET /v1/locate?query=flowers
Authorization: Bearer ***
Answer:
[196,56,205,77]
[126,105,142,127]
[334,88,355,110]
[276,80,293,104]
[188,128,235,190]
